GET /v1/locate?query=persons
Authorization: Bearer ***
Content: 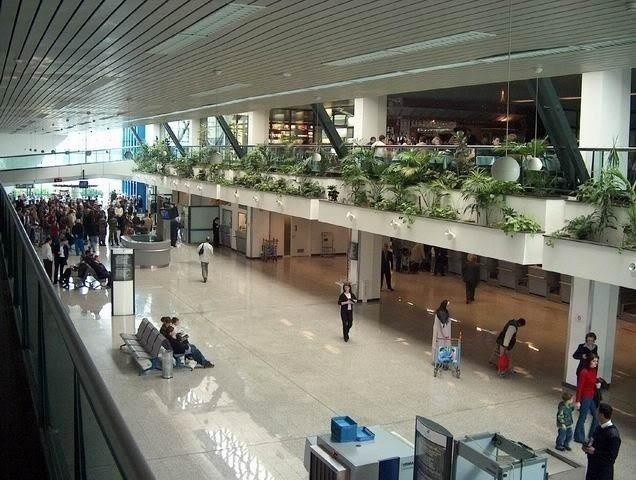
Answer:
[367,134,556,192]
[213,218,220,248]
[574,353,602,444]
[462,254,479,304]
[338,283,357,340]
[555,392,577,451]
[382,244,394,291]
[432,300,451,370]
[489,318,525,371]
[16,190,182,285]
[390,239,446,276]
[196,236,213,282]
[572,333,598,384]
[582,403,621,480]
[160,317,215,369]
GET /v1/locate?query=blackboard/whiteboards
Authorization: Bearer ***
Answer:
[322,232,333,247]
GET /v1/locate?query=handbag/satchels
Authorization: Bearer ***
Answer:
[199,243,204,255]
[497,349,509,370]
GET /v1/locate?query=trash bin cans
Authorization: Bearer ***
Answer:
[161,351,173,378]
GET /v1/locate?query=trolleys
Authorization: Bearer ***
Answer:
[433,329,463,380]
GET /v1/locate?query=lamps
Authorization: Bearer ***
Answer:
[491,0,520,181]
[210,70,223,165]
[526,68,543,171]
[313,95,322,162]
[24,97,134,159]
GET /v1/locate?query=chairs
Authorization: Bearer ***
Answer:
[120,317,193,376]
[71,258,110,291]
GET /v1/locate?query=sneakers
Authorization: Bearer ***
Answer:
[184,357,215,370]
[556,444,569,452]
[574,437,589,443]
[344,334,350,342]
[204,278,207,283]
[387,286,394,291]
[466,296,475,304]
